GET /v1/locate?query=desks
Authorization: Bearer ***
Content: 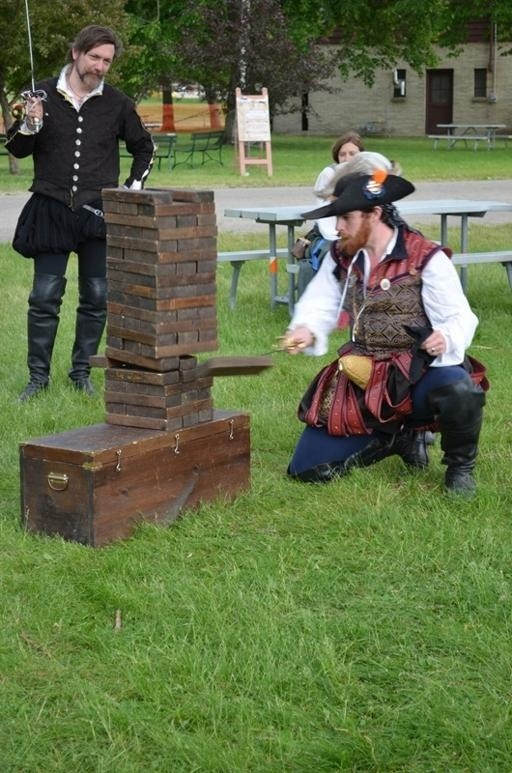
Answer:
[224,198,512,319]
[437,124,505,148]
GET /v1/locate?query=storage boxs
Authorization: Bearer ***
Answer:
[19,412,253,547]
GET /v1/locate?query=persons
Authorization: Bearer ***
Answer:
[5,24,158,405]
[279,151,491,493]
[311,132,368,266]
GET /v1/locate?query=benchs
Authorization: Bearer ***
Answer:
[215,247,298,310]
[485,135,512,141]
[428,135,488,151]
[119,135,178,172]
[449,250,512,294]
[176,128,226,169]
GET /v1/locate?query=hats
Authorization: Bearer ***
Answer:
[300,172,415,220]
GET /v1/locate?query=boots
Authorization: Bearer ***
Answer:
[68,280,107,400]
[288,431,435,482]
[18,275,67,403]
[435,389,485,502]
